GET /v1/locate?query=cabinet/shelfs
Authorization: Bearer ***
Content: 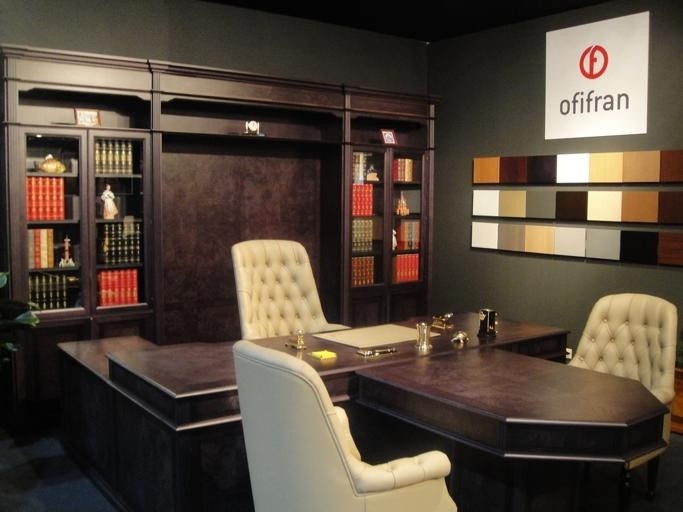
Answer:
[351,145,424,287]
[16,125,147,314]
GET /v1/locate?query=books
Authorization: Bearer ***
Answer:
[23,139,140,310]
[351,150,421,286]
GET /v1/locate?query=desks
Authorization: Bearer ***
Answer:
[54,311,660,501]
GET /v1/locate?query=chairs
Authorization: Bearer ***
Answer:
[231,240,352,341]
[230,338,458,511]
[556,292,676,500]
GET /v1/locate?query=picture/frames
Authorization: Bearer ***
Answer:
[380,129,397,146]
[73,107,102,125]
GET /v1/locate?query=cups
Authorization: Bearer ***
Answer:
[479,308,495,338]
[415,322,431,353]
